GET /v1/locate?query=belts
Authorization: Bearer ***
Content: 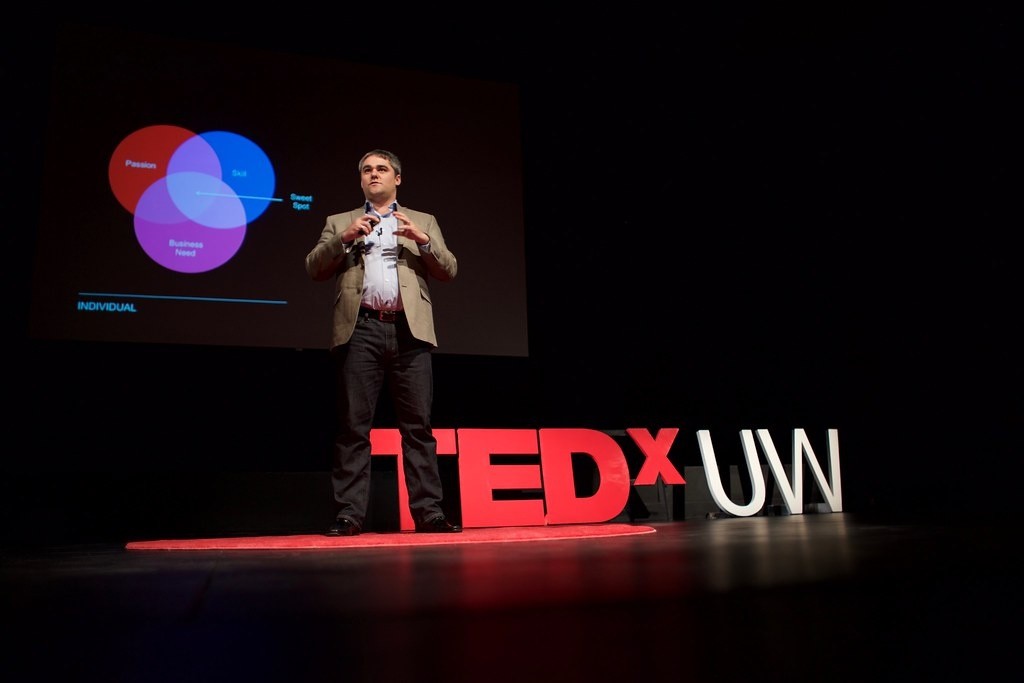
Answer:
[359,308,406,322]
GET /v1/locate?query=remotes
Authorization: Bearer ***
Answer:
[357,216,382,235]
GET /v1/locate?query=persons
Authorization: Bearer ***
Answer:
[305,149,463,536]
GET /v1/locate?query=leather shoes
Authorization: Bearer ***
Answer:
[417,516,462,532]
[328,518,359,536]
